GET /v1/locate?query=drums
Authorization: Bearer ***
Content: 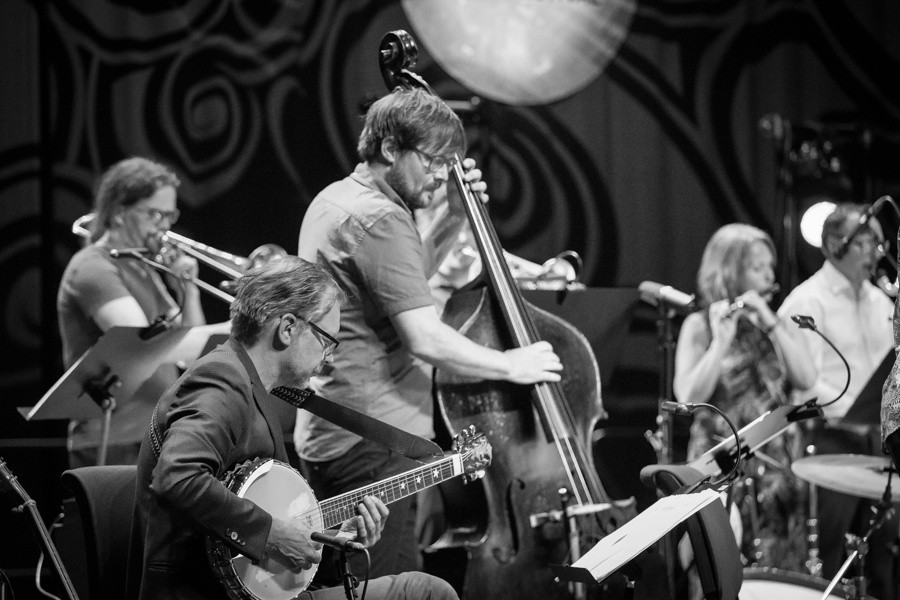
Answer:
[738,566,878,600]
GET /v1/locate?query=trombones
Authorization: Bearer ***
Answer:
[71,209,253,303]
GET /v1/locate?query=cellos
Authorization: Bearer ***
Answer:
[378,31,670,600]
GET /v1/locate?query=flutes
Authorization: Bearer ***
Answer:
[715,283,780,322]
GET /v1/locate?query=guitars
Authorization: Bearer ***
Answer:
[206,424,493,600]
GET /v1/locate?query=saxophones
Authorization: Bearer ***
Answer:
[869,264,899,297]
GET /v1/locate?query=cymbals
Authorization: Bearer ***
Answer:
[791,453,900,503]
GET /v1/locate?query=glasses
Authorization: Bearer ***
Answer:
[133,207,180,226]
[295,316,339,356]
[409,147,457,174]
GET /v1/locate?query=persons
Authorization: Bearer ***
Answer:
[672,203,900,600]
[129,257,461,600]
[292,87,585,588]
[58,157,234,467]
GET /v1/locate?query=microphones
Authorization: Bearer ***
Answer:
[638,280,695,309]
[310,532,365,552]
[833,197,889,260]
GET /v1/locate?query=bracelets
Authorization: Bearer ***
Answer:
[764,317,781,335]
